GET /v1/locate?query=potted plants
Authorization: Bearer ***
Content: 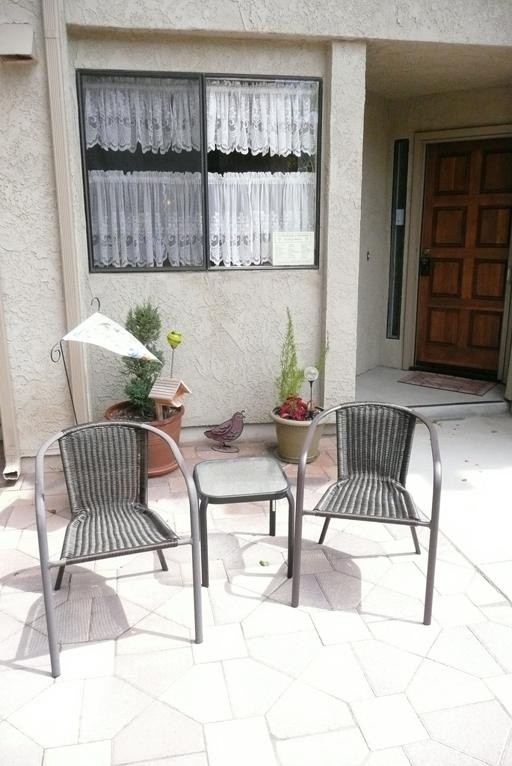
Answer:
[270,307,329,463]
[105,299,191,478]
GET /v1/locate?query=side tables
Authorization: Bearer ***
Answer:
[193,455,292,587]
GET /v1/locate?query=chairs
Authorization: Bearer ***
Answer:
[292,401,442,624]
[35,421,203,677]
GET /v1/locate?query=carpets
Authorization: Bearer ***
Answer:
[398,371,497,396]
